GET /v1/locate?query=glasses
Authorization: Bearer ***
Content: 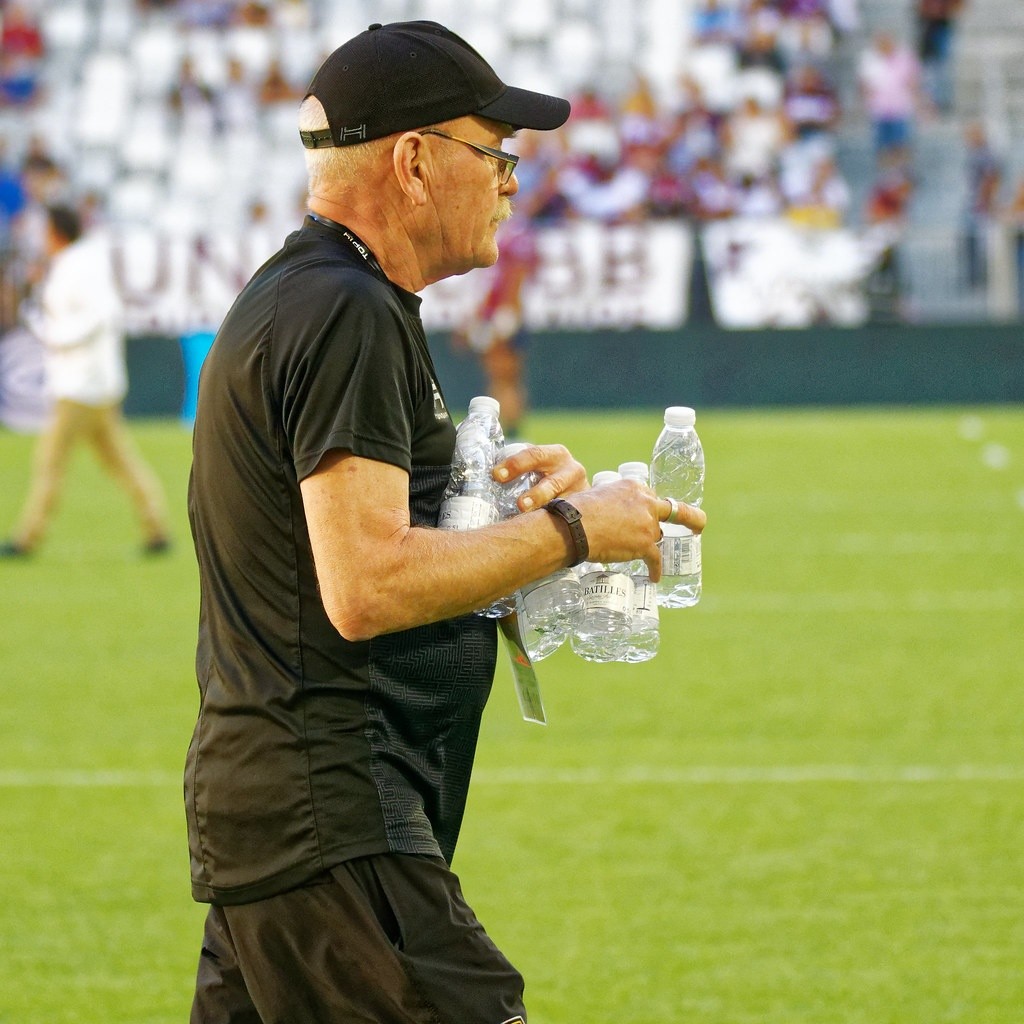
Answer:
[414,129,520,185]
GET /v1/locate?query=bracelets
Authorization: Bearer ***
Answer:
[541,497,591,568]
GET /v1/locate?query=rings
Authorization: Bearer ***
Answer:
[656,528,664,547]
[664,497,678,524]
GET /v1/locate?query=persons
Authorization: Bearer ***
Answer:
[0,1,1024,316]
[0,208,167,560]
[179,21,706,1024]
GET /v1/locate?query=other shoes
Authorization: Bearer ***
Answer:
[1,539,41,560]
[145,531,170,554]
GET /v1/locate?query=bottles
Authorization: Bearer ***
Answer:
[515,592,568,663]
[649,407,706,608]
[619,462,659,663]
[501,443,585,634]
[570,471,633,664]
[438,395,508,529]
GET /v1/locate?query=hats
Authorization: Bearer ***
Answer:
[300,19,572,148]
